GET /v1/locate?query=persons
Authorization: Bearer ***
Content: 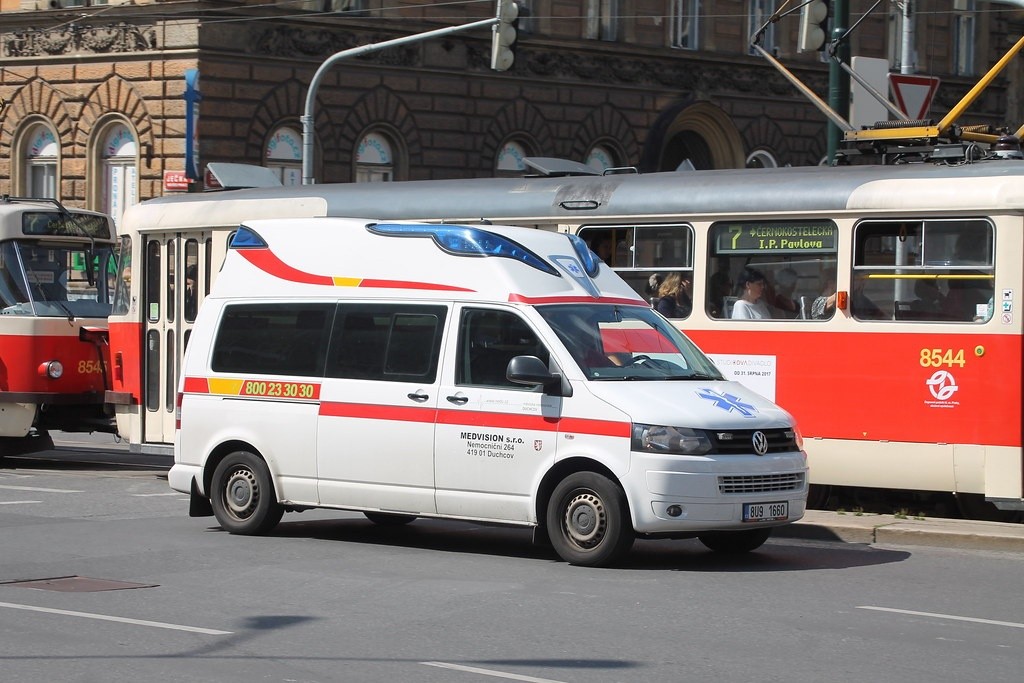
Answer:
[656,272,692,317]
[811,272,866,319]
[573,321,618,368]
[36,256,72,300]
[910,279,946,317]
[883,249,895,265]
[123,239,160,399]
[711,255,797,319]
[590,236,612,262]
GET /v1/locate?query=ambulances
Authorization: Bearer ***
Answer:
[166,216,809,569]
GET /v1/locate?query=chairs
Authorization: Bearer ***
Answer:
[650,297,659,308]
[800,295,813,320]
[722,295,740,318]
[895,301,914,320]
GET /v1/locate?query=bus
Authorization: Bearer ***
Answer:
[0,201,119,437]
[105,161,1024,522]
